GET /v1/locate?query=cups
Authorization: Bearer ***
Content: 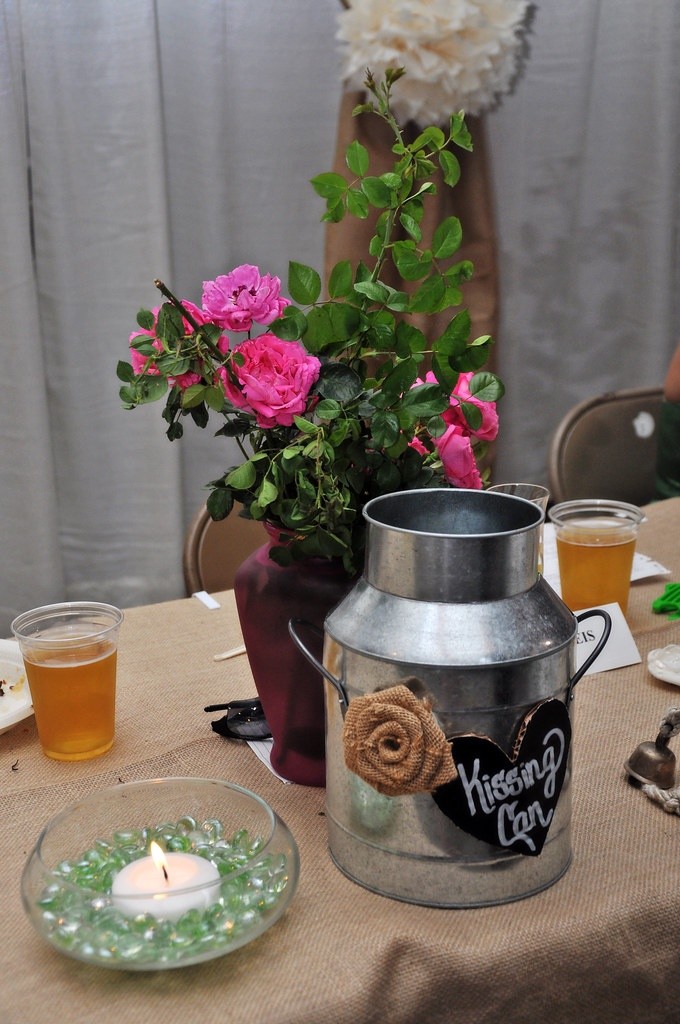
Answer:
[11,601,123,760]
[486,484,549,577]
[548,500,645,617]
[287,487,610,907]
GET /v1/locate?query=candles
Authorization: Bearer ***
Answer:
[108,839,225,921]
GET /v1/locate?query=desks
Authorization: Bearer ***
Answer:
[0,494,680,1024]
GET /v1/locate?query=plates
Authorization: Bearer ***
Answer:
[0,639,34,734]
[647,644,680,686]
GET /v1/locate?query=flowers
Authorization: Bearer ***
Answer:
[335,0,537,131]
[113,67,506,547]
[342,684,458,801]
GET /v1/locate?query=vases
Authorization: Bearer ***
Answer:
[233,520,366,787]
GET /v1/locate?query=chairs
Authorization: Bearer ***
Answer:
[184,497,269,599]
[548,384,680,520]
